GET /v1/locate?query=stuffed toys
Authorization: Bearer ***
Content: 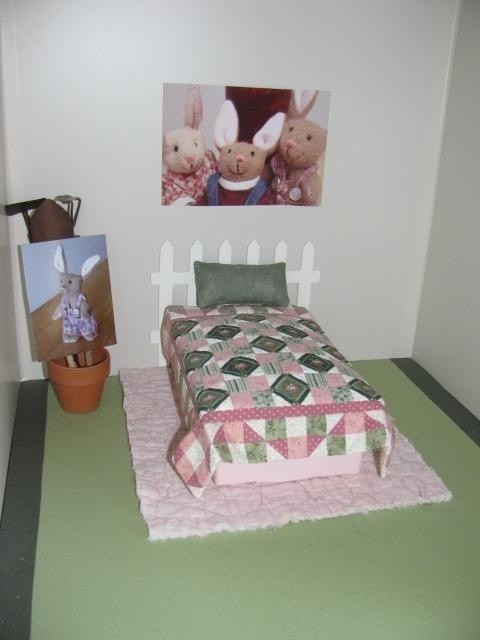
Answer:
[261,90,329,207]
[206,99,287,205]
[162,84,220,205]
[50,245,101,345]
